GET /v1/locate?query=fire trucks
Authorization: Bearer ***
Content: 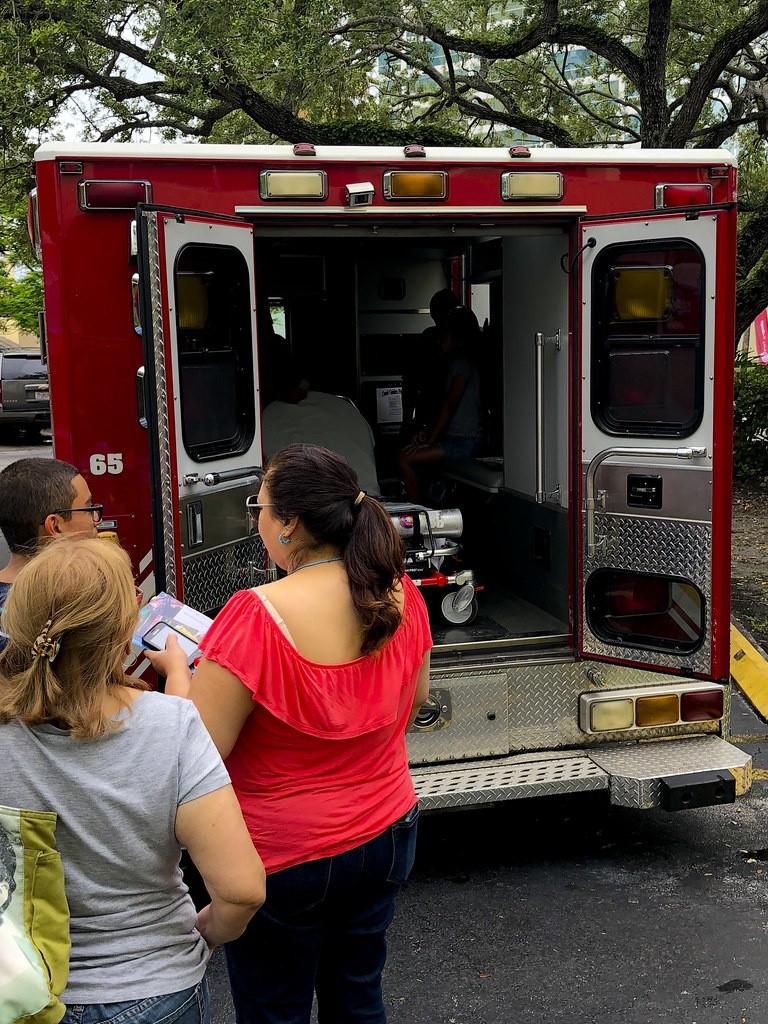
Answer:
[22,134,767,822]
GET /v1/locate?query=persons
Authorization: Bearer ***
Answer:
[0,457,103,653]
[144,444,433,1024]
[0,537,267,1024]
[259,288,500,504]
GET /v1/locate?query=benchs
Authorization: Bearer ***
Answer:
[436,461,504,504]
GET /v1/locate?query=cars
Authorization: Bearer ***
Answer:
[0,347,50,434]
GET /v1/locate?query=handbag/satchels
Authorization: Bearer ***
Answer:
[0,806,70,1024]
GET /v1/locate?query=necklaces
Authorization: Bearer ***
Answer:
[288,556,344,575]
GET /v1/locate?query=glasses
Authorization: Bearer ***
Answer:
[40,504,104,525]
[247,494,278,523]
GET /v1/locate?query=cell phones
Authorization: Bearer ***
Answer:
[143,622,203,669]
